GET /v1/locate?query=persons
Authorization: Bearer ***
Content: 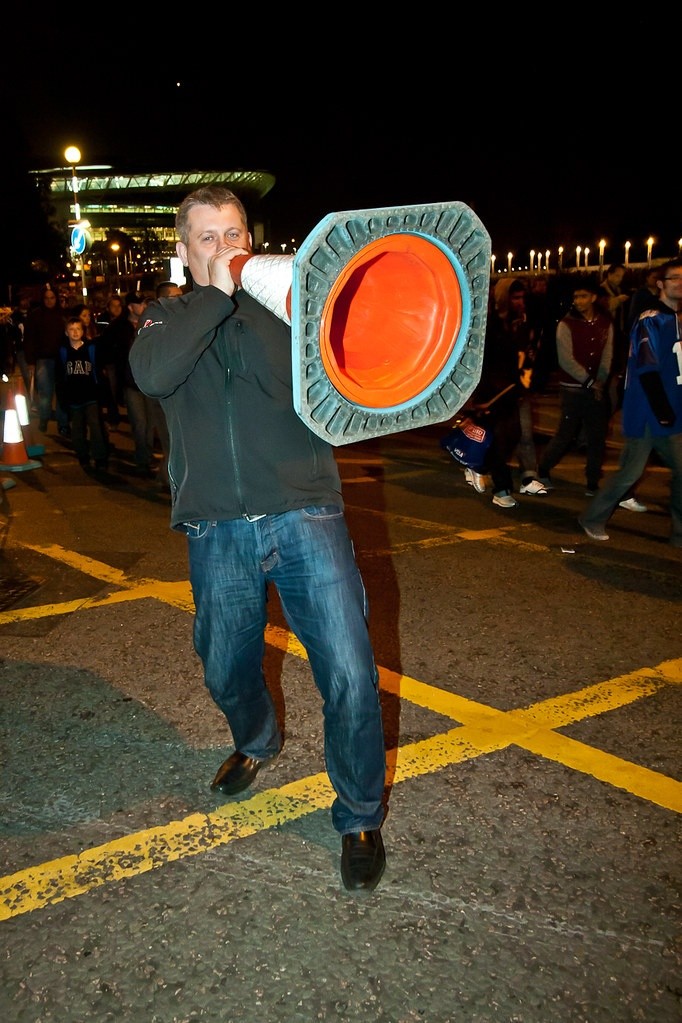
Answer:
[131,184,383,900]
[464,258,682,548]
[0,282,182,486]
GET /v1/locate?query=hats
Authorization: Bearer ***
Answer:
[124,291,154,305]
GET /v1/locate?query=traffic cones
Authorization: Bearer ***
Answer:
[9,364,45,458]
[0,382,43,472]
[226,200,490,447]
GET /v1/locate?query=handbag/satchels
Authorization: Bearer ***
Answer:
[439,411,497,474]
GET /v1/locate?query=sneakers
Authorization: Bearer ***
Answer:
[466,468,487,493]
[518,480,546,494]
[492,494,517,508]
[619,496,648,513]
[670,531,682,549]
[579,517,609,540]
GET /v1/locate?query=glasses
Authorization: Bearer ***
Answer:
[661,274,682,283]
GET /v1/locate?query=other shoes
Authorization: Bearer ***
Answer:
[585,485,599,498]
[39,421,47,432]
[540,472,554,492]
[96,465,108,473]
[82,464,94,475]
[150,463,159,472]
[58,426,70,436]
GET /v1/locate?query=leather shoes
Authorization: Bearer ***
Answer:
[211,751,262,795]
[339,828,387,896]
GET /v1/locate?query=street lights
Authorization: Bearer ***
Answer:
[599,239,607,283]
[507,252,513,273]
[537,252,543,273]
[647,236,654,270]
[545,249,551,272]
[529,249,535,272]
[624,240,632,269]
[677,238,682,256]
[63,146,88,307]
[490,254,496,274]
[111,243,121,297]
[575,246,582,268]
[583,247,590,268]
[558,246,564,272]
